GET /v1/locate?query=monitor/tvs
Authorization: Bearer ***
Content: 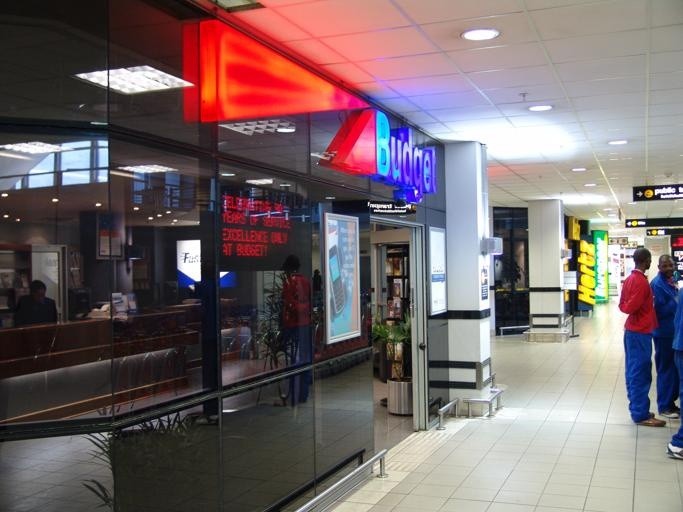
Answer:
[68,286,92,319]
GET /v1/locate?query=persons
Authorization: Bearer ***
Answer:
[617,248,667,427]
[11,280,58,353]
[664,286,683,461]
[273,255,313,408]
[649,253,681,419]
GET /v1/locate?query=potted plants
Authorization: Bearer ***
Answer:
[369,308,414,417]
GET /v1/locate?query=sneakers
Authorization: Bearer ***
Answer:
[636,408,683,459]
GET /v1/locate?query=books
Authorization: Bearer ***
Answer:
[385,257,408,317]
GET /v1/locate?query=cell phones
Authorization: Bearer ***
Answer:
[329,245,346,316]
